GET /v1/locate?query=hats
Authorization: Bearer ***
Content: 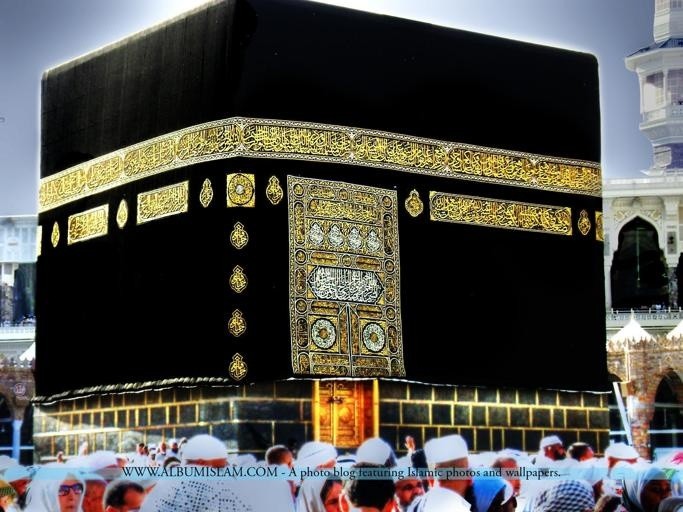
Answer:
[542,436,562,448]
[356,439,392,465]
[424,434,468,463]
[182,435,226,461]
[297,441,337,470]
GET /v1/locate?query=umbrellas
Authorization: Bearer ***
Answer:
[666,319,683,341]
[18,342,36,362]
[606,318,657,345]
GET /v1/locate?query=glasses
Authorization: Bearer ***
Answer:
[58,483,82,496]
